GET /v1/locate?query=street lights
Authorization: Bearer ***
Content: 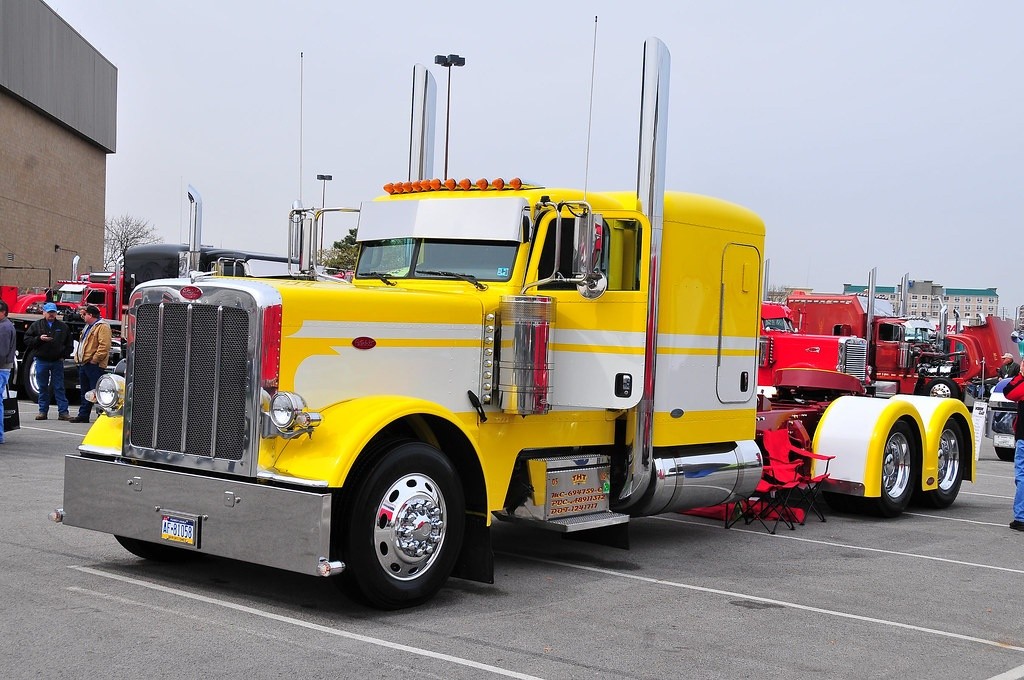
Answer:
[432,53,466,181]
[316,174,333,265]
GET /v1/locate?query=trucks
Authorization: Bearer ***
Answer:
[0,32,1023,613]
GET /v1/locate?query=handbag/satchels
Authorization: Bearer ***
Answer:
[2,383,20,432]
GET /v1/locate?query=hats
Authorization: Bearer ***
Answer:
[1001,352,1013,359]
[85,306,100,315]
[43,302,57,312]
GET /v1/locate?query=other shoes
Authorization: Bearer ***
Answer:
[58,414,74,420]
[35,413,48,420]
[69,414,90,423]
[1009,520,1024,532]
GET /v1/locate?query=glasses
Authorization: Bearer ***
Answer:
[83,312,91,315]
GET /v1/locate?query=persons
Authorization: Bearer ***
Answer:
[1003,358,1024,532]
[998,352,1020,381]
[24,302,74,421]
[69,305,112,423]
[0,300,16,444]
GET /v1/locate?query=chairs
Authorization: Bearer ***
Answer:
[724,430,836,535]
